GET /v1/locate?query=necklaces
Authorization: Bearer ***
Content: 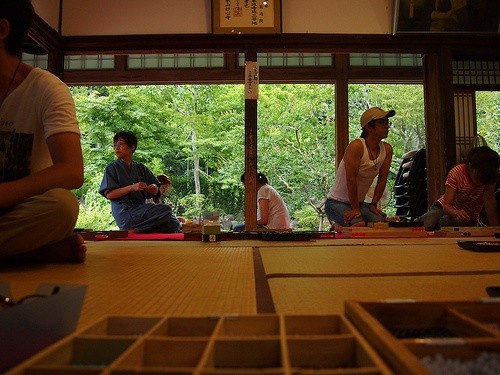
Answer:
[364,137,378,171]
[0,59,22,108]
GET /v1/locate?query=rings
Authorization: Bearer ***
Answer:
[456,213,458,216]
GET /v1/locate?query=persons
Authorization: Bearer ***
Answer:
[144,173,171,205]
[0,0,87,266]
[98,130,180,233]
[324,107,396,234]
[414,145,500,231]
[233,171,290,231]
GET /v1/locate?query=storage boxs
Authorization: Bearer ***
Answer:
[200,225,221,242]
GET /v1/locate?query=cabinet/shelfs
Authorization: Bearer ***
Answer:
[392,147,427,227]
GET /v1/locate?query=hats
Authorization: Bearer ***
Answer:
[360,106,397,127]
